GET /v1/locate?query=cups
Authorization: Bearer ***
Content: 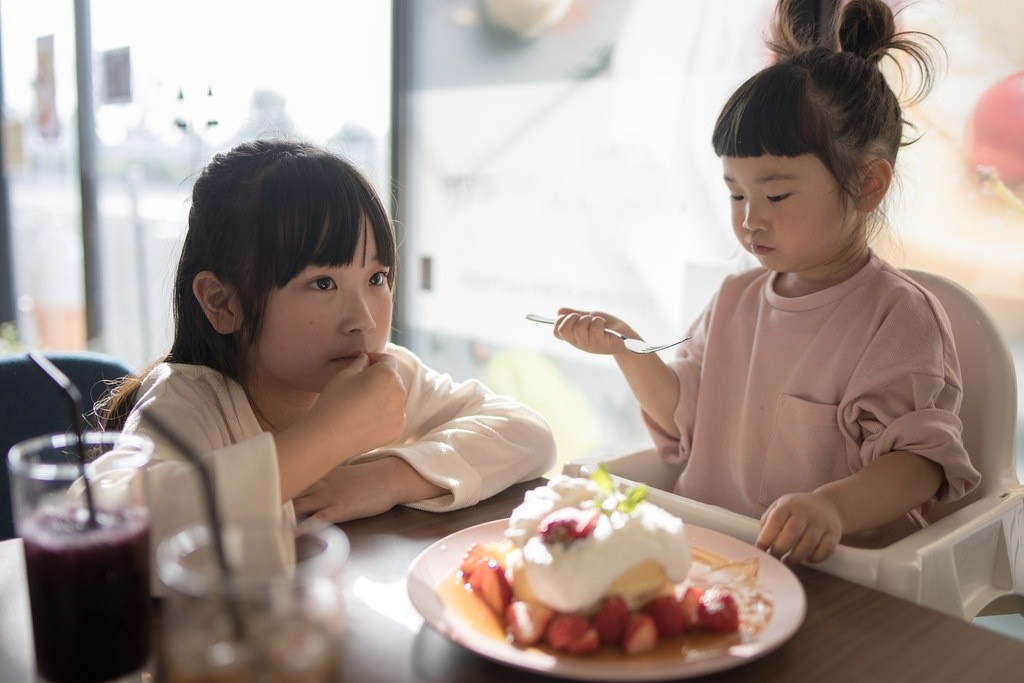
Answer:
[8,429,158,683]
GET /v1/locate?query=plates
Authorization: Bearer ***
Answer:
[405,517,807,682]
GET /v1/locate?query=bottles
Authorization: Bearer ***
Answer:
[155,514,352,683]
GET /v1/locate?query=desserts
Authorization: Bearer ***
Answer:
[501,459,690,615]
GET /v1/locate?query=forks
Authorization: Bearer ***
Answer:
[524,312,693,355]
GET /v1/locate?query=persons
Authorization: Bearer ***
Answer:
[65,137,557,540]
[553,1,982,566]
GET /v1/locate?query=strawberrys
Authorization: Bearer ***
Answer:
[462,542,740,656]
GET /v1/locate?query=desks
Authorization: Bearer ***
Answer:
[127,475,1024,683]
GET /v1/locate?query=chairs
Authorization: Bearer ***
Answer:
[557,266,1024,621]
[0,352,139,545]
